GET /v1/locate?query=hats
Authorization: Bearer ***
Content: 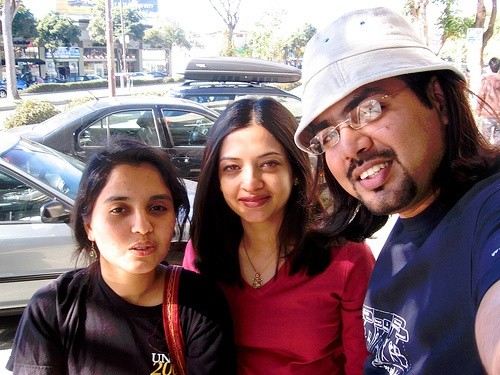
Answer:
[294,7,467,155]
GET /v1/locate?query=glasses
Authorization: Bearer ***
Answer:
[306,81,419,155]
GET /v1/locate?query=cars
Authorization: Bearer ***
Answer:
[0,96,223,182]
[42,71,171,86]
[0,77,28,98]
[165,99,304,135]
[0,132,199,322]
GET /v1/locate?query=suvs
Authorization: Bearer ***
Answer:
[170,54,303,103]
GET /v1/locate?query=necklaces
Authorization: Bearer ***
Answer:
[242,239,277,289]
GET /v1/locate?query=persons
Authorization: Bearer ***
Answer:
[294,7,500,375]
[5,141,237,375]
[476,57,500,147]
[182,98,376,375]
